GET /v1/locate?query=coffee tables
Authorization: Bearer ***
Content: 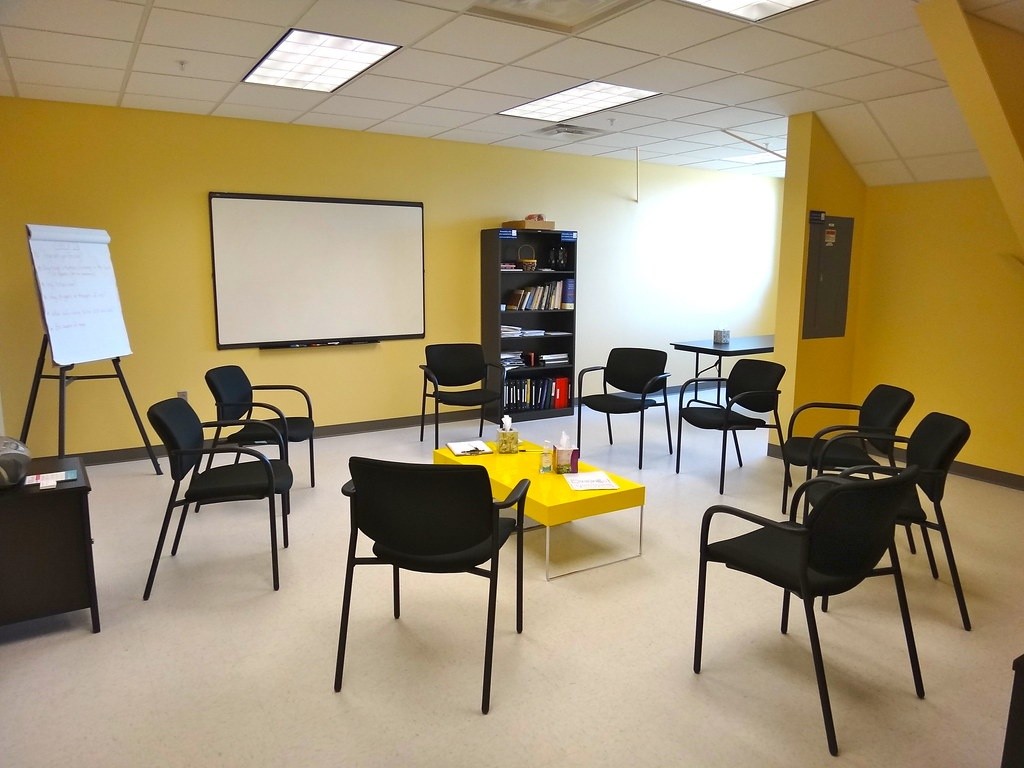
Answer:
[431,437,645,581]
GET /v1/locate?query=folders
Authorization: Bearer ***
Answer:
[503,378,573,412]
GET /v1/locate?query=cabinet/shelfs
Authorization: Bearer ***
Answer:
[478,227,579,425]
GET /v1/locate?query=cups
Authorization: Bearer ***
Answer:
[526,352,534,367]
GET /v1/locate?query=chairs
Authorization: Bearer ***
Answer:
[334,456,530,714]
[417,343,505,451]
[577,347,671,470]
[142,396,294,603]
[676,357,793,495]
[192,365,315,514]
[789,413,972,632]
[781,381,915,515]
[692,462,927,756]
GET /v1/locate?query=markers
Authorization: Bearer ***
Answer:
[310,344,327,347]
[290,345,307,348]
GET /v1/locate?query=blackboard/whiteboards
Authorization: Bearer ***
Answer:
[209,191,425,350]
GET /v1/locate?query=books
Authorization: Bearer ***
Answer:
[501,279,575,311]
[24,469,78,485]
[504,377,552,411]
[500,350,569,367]
[501,263,523,271]
[500,325,572,338]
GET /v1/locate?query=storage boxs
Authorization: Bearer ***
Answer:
[502,220,554,229]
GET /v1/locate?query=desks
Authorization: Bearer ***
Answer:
[669,335,775,404]
[0,454,101,635]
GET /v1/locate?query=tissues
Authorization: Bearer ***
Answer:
[552,430,580,474]
[714,329,730,344]
[496,414,520,454]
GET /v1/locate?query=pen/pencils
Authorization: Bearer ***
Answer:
[519,449,543,452]
[461,450,484,453]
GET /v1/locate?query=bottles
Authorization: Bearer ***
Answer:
[541,440,551,472]
[549,244,566,270]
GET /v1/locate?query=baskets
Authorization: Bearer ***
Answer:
[516,244,537,271]
